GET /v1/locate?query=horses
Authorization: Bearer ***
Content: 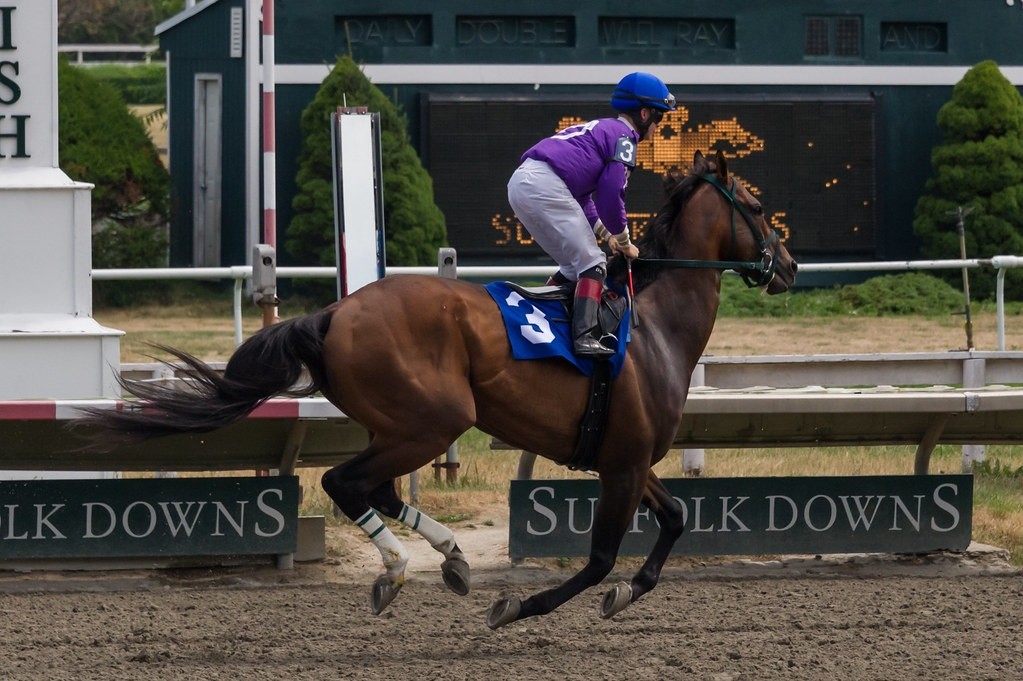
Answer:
[51,149,799,629]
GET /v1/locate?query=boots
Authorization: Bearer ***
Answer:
[571,265,617,356]
[545,276,559,286]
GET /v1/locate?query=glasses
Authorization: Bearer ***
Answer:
[665,91,675,110]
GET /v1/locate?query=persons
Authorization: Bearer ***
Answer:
[507,71,675,358]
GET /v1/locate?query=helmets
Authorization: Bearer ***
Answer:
[611,73,675,113]
